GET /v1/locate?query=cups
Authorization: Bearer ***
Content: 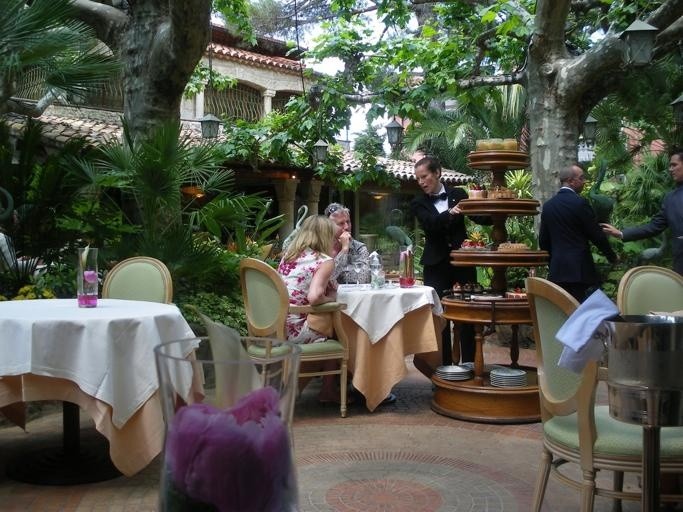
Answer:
[325,205,340,217]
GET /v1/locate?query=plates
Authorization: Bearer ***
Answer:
[432,136,554,422]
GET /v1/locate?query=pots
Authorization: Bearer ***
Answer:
[587,308,683,428]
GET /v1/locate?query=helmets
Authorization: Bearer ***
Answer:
[436,365,471,382]
[489,370,527,389]
[458,248,491,250]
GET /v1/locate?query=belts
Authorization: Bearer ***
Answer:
[341,254,353,290]
[351,254,365,288]
[369,254,380,290]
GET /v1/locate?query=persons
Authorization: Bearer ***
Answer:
[597,149,683,277]
[0,208,44,274]
[538,165,621,304]
[276,216,355,403]
[414,158,492,366]
[324,203,371,284]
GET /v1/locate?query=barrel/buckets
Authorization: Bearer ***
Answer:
[319,395,356,407]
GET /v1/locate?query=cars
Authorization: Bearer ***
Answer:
[371,271,385,287]
[153,339,299,512]
[488,138,502,148]
[476,139,487,150]
[398,246,415,287]
[503,138,516,150]
[77,246,99,308]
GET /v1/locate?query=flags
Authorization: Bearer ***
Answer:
[75,244,100,309]
[399,245,415,288]
[153,332,305,510]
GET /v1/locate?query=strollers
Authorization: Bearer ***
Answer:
[430,193,447,203]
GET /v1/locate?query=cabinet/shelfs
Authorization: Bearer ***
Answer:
[453,281,484,293]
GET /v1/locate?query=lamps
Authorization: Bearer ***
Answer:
[615,264,683,314]
[100,256,175,303]
[239,255,353,418]
[518,276,682,511]
